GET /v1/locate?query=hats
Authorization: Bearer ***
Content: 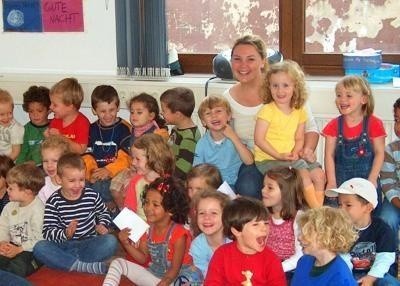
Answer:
[324,177,379,210]
